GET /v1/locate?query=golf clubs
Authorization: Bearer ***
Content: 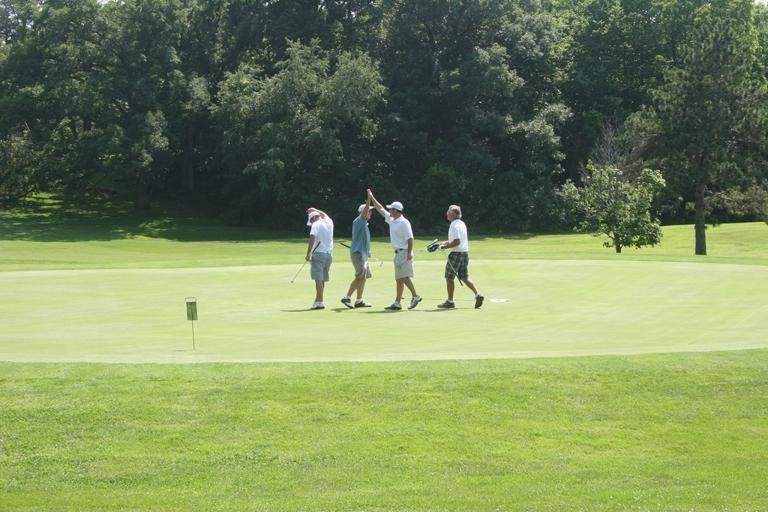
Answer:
[339,241,383,267]
[427,243,463,286]
[291,241,321,283]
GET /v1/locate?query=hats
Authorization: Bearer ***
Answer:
[386,202,403,212]
[307,211,320,226]
[358,205,373,213]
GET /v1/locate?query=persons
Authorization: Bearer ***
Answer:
[340,188,375,309]
[366,188,423,311]
[427,204,485,309]
[304,207,335,309]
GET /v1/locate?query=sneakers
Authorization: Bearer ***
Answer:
[355,300,371,307]
[475,296,483,309]
[311,301,325,308]
[384,304,402,310]
[438,300,454,308]
[341,299,353,308]
[408,296,422,309]
